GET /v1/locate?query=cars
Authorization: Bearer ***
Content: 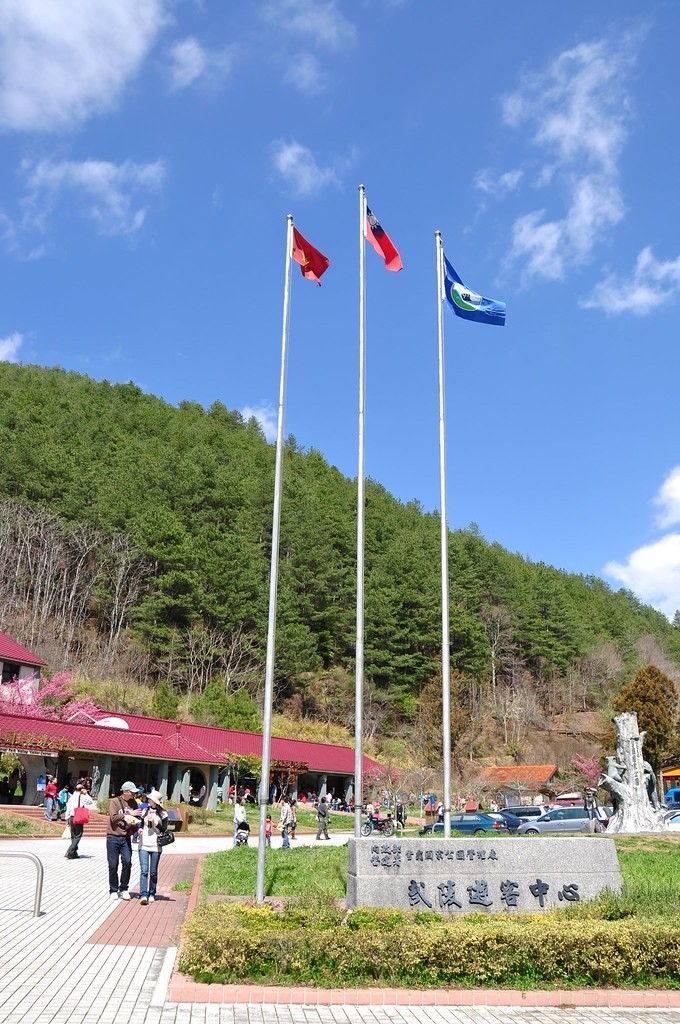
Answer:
[484,811,530,833]
[418,813,509,836]
[597,806,613,822]
[648,801,680,832]
[545,805,564,818]
[516,807,601,835]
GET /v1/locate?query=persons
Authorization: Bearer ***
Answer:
[137,791,168,904]
[367,801,380,818]
[325,792,355,813]
[271,785,276,804]
[83,777,93,793]
[233,797,246,845]
[316,797,330,840]
[133,777,158,843]
[64,784,93,859]
[392,800,405,828]
[0,777,25,804]
[298,791,320,806]
[188,783,206,807]
[228,786,255,804]
[568,803,577,818]
[490,801,498,812]
[548,804,554,813]
[436,803,443,823]
[45,777,71,822]
[107,782,142,900]
[265,795,297,848]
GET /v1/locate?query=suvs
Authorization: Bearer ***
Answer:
[500,804,550,822]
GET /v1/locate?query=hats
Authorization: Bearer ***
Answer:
[147,790,162,805]
[121,781,139,792]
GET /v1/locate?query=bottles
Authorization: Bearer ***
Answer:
[148,815,152,828]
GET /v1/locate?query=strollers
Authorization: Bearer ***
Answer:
[235,822,250,847]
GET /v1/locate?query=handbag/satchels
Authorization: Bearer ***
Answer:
[62,825,71,839]
[73,793,89,824]
[157,828,175,846]
[277,822,285,831]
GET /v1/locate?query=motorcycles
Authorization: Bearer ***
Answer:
[359,811,394,837]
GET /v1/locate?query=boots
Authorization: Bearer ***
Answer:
[316,833,323,839]
[324,833,331,839]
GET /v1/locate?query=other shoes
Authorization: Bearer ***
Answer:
[120,891,131,900]
[111,892,118,899]
[149,896,154,902]
[65,853,80,859]
[140,897,147,905]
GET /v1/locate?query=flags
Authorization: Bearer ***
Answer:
[292,226,330,287]
[365,206,403,272]
[443,252,507,326]
